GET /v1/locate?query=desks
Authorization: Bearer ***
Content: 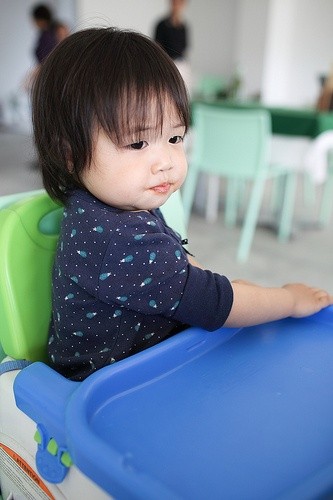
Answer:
[201,103,333,243]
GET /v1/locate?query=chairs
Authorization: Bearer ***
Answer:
[182,101,298,263]
[0,189,333,500]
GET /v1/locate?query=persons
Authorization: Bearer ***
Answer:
[153,0,192,83]
[27,24,333,383]
[18,2,70,172]
